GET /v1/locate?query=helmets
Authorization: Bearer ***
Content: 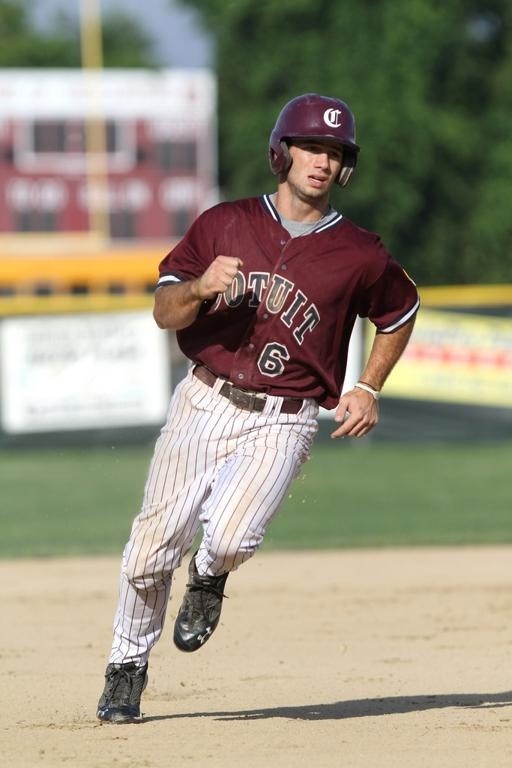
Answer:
[268,93,361,187]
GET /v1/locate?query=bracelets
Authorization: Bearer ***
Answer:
[358,381,376,391]
[354,383,380,401]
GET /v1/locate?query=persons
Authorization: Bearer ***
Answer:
[96,90,421,724]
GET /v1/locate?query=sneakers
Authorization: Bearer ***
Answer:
[96,660,148,722]
[174,547,230,651]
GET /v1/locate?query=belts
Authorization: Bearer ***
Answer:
[193,365,303,415]
